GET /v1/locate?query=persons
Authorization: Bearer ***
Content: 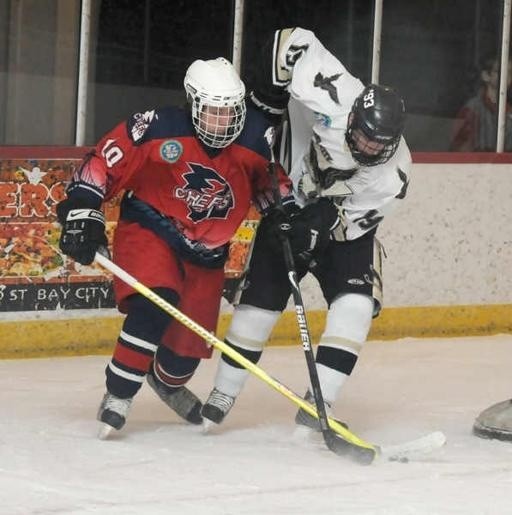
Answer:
[447,54,512,153]
[201,27,413,435]
[58,55,307,439]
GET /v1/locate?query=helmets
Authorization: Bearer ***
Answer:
[184,56,247,109]
[355,84,407,145]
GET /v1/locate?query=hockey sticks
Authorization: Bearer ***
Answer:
[265,125,375,465]
[92,251,446,460]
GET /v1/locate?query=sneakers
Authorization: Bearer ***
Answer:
[198,387,236,426]
[294,390,349,433]
[146,372,203,426]
[96,393,134,431]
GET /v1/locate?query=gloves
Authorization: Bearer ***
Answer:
[261,195,340,270]
[55,195,111,264]
